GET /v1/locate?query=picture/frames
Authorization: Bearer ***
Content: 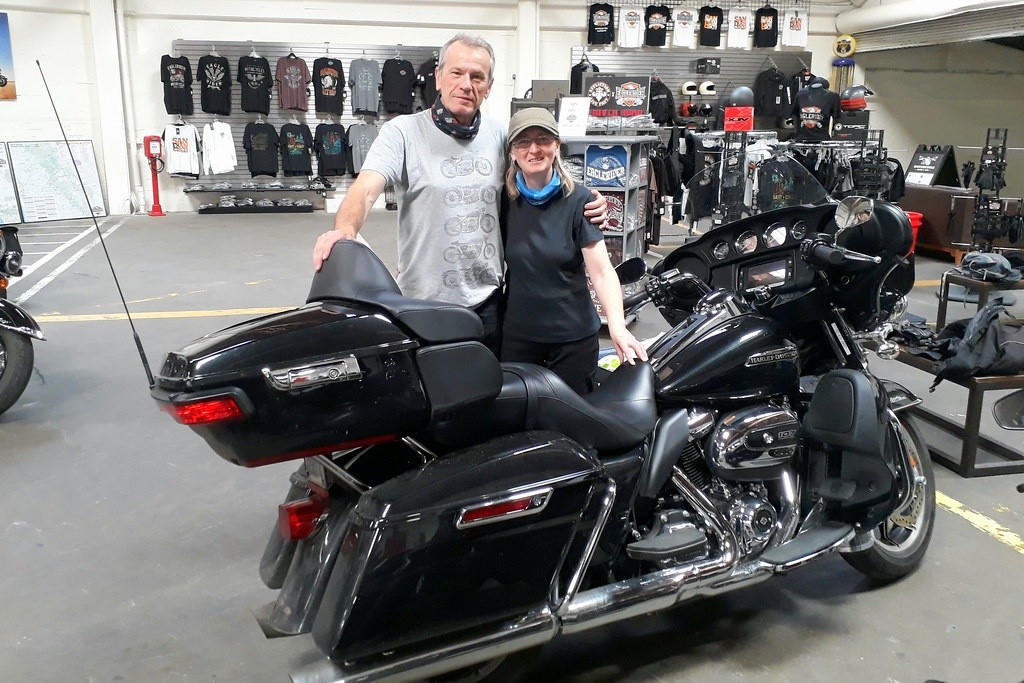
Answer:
[0,141,23,226]
[6,140,108,223]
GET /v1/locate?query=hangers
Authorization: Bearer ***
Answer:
[759,61,820,80]
[631,130,900,165]
[590,0,806,11]
[161,40,441,68]
[571,52,669,90]
[164,113,377,131]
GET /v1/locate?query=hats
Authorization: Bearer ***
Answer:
[506,107,559,144]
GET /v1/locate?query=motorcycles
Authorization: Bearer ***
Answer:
[36,58,936,682]
[0,222,47,416]
[0,69,7,87]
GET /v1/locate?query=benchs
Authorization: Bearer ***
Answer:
[935,270,1024,332]
[849,313,1024,479]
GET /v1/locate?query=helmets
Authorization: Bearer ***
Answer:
[682,80,698,95]
[699,103,712,116]
[838,84,874,111]
[699,81,717,95]
[681,102,698,117]
[780,116,795,129]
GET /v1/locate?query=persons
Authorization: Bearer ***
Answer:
[497,104,649,396]
[311,31,508,361]
